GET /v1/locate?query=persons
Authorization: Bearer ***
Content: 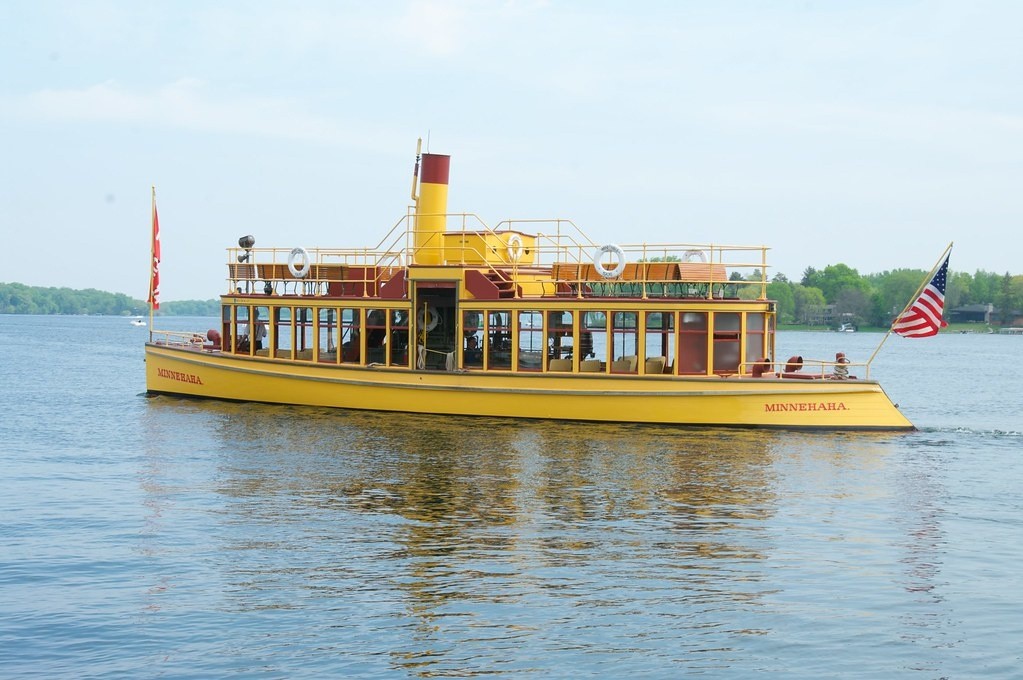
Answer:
[234,308,267,352]
[465,337,483,361]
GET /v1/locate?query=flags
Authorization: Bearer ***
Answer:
[888,248,950,339]
[145,204,163,310]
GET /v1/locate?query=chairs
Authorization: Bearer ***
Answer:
[549,355,666,373]
[256,348,313,361]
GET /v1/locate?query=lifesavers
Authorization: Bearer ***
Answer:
[416,306,439,333]
[592,242,627,278]
[681,248,709,263]
[287,247,310,278]
[507,234,523,261]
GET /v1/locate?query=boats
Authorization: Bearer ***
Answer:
[145,132,919,432]
[131,319,146,327]
